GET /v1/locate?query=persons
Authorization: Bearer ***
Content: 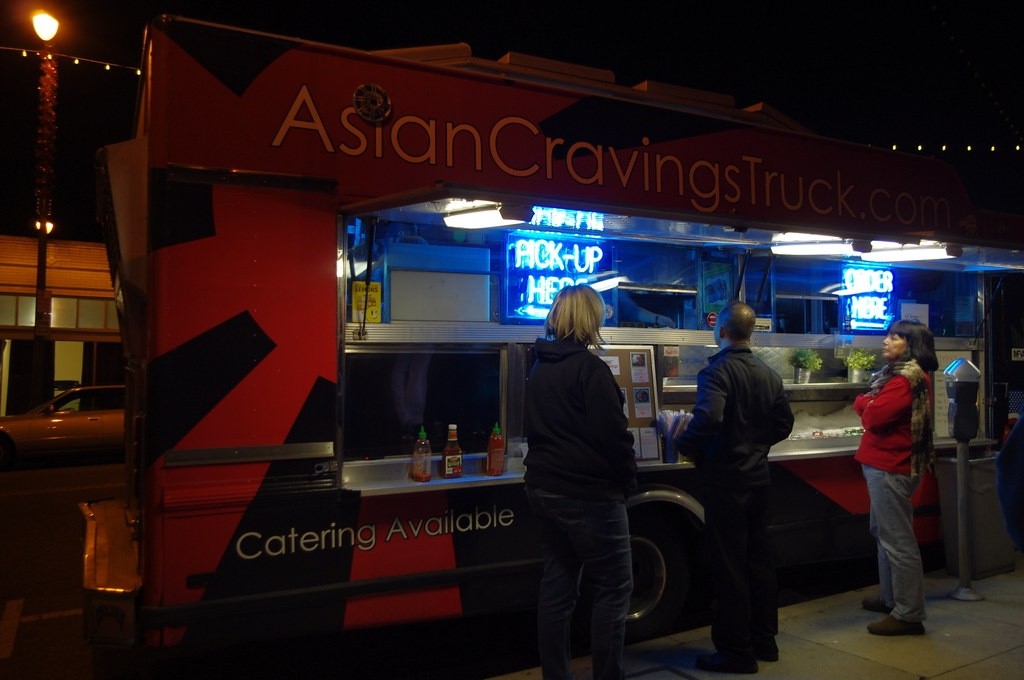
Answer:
[852,319,939,638]
[669,302,795,676]
[514,284,641,679]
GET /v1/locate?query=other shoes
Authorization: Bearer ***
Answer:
[867,612,925,636]
[695,652,758,674]
[749,644,779,663]
[862,597,894,613]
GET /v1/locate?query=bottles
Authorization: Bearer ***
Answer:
[486,422,504,476]
[412,426,431,481]
[442,423,464,479]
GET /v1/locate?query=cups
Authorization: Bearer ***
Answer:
[660,437,678,463]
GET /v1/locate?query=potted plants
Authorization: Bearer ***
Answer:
[789,349,822,384]
[844,349,878,383]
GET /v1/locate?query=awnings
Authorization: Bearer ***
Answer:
[343,177,1024,274]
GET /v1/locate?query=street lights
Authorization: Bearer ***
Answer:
[28,2,71,411]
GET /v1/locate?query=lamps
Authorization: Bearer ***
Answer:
[442,205,524,230]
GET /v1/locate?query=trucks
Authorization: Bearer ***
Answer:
[74,13,1024,657]
